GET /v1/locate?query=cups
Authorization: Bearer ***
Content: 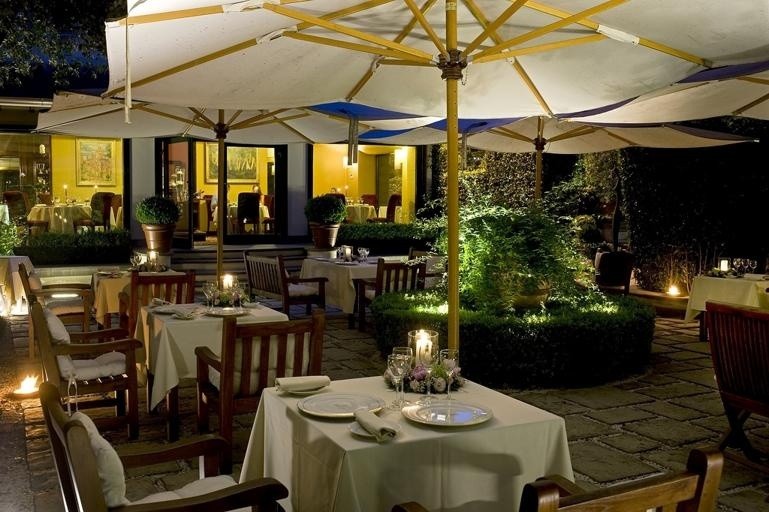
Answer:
[717,257,732,274]
[407,328,440,370]
[146,250,160,272]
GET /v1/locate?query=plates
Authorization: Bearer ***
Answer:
[335,261,377,265]
[98,271,128,275]
[401,400,493,427]
[152,301,260,320]
[347,418,402,437]
[297,391,387,418]
[289,386,327,397]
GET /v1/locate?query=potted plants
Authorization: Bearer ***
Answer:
[543,148,642,293]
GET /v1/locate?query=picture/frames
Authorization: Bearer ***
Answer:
[204,141,260,184]
[75,135,118,187]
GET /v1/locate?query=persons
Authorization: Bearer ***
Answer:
[210,184,234,235]
[251,184,265,207]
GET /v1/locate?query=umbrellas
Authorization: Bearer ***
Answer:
[310,102,529,171]
[458,116,761,213]
[100,0,769,370]
[30,87,376,291]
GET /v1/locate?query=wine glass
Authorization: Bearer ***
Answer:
[54,196,61,206]
[386,345,460,410]
[336,247,370,264]
[130,252,143,267]
[201,279,246,314]
[733,257,758,274]
